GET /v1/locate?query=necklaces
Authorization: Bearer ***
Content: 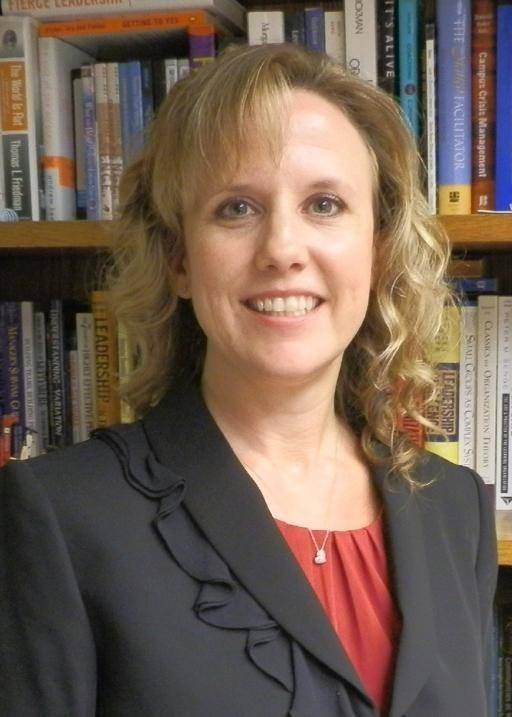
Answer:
[230,440,344,565]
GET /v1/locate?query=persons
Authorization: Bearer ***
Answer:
[0,41,500,716]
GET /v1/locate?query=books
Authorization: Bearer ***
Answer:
[1,285,177,467]
[0,0,512,224]
[392,249,512,511]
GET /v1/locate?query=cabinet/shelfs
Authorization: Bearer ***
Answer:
[0,0,511,565]
